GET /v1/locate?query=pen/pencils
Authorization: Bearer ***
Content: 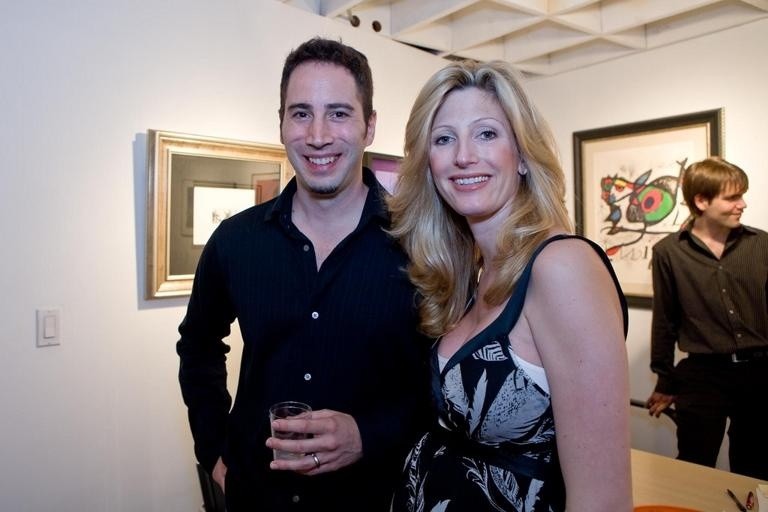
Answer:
[727,489,747,512]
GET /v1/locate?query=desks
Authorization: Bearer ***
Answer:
[630,447,768,512]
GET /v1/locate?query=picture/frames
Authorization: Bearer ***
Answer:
[571,106,727,310]
[144,128,296,302]
[362,151,405,198]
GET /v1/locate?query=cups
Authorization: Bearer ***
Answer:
[268,402,313,460]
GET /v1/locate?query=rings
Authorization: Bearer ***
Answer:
[311,453,320,467]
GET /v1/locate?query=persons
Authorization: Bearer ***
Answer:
[176,39,474,512]
[380,58,635,511]
[644,155,768,480]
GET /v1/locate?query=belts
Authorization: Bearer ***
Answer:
[689,350,768,367]
